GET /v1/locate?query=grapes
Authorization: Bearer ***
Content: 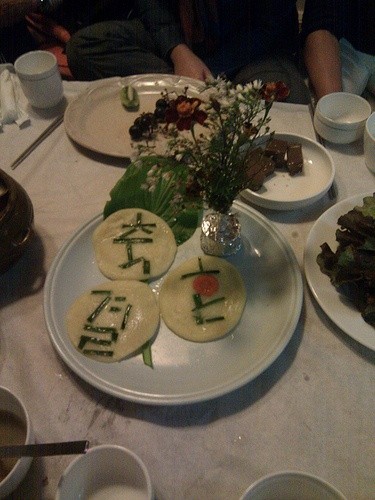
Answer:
[129,99,171,138]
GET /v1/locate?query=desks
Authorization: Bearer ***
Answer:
[0,72,375,500]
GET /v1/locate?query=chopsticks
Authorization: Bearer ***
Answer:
[9,113,64,170]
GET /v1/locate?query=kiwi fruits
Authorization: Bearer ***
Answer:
[121,85,139,108]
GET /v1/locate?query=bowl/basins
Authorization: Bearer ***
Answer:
[314,92,371,144]
[0,385,37,498]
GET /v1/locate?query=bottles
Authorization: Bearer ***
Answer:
[0,169,33,272]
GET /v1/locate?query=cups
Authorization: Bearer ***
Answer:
[56,444,155,500]
[240,470,347,500]
[363,111,375,175]
[15,50,64,108]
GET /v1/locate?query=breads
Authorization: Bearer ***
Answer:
[245,138,304,192]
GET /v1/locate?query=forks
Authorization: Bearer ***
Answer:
[303,78,338,200]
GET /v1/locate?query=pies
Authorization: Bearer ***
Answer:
[93,208,177,282]
[158,256,246,343]
[65,278,160,362]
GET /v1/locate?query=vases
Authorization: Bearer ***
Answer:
[200,212,242,256]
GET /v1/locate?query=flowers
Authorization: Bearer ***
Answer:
[143,70,294,215]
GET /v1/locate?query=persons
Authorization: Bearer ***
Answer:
[299,0,375,102]
[65,0,312,106]
[25,0,138,79]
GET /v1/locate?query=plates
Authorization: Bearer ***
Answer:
[303,190,375,353]
[65,73,238,156]
[41,199,304,405]
[238,132,335,210]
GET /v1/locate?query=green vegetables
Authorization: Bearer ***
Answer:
[317,191,375,328]
[102,156,204,370]
